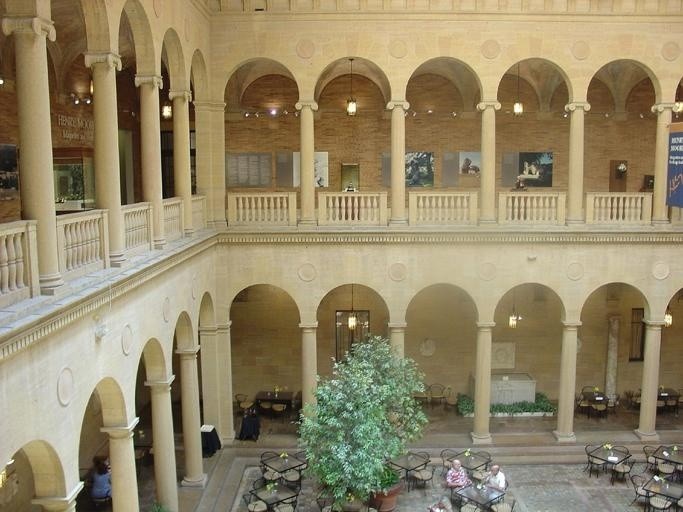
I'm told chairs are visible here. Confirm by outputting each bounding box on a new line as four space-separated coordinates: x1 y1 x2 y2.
239 448 515 512
87 441 154 512
584 442 683 511
572 384 682 423
417 380 447 410
234 392 287 420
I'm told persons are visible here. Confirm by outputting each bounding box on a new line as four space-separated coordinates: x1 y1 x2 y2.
428 495 454 512
445 459 472 493
481 464 506 492
87 455 112 499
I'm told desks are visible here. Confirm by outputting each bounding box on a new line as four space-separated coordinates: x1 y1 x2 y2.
257 390 293 411
132 426 153 451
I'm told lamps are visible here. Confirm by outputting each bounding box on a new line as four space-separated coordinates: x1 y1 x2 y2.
346 56 358 120
347 284 358 331
507 288 519 329
511 59 524 120
